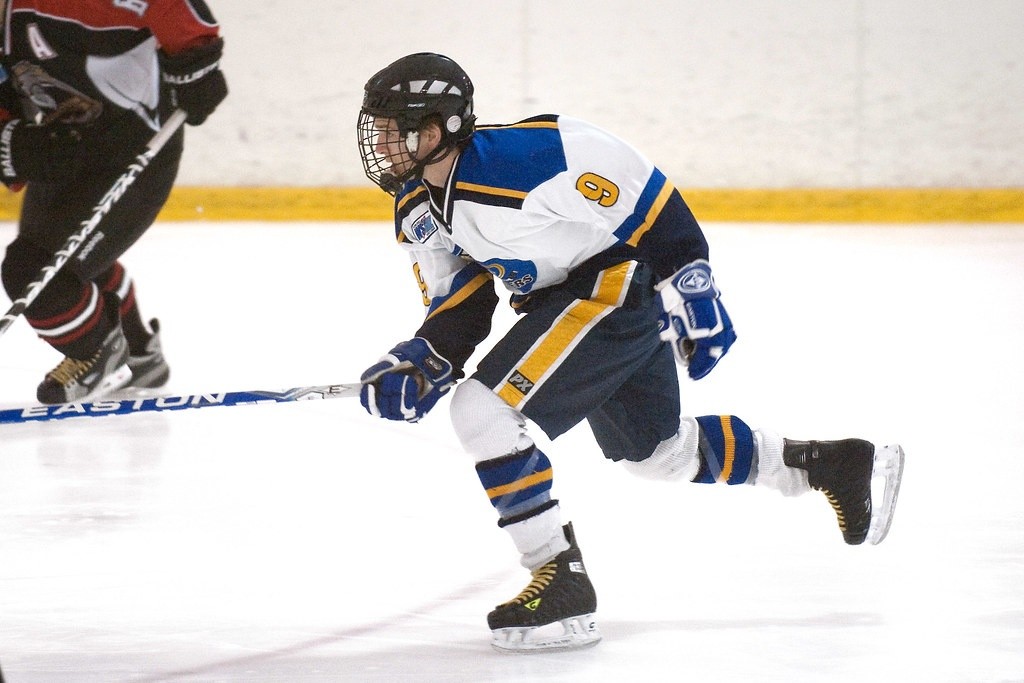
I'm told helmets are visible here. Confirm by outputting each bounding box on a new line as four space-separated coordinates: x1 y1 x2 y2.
356 52 477 194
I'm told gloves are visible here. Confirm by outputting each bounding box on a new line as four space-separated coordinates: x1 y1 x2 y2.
359 338 464 422
651 258 737 381
0 116 81 184
154 36 227 126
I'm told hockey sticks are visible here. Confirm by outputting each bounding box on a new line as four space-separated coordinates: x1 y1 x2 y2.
1 97 190 348
0 376 424 426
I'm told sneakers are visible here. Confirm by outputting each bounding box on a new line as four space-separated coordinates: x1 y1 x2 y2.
488 520 602 651
96 316 170 401
782 438 907 543
37 291 144 406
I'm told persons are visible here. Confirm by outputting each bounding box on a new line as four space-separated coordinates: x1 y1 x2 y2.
0 0 230 407
356 51 905 652
